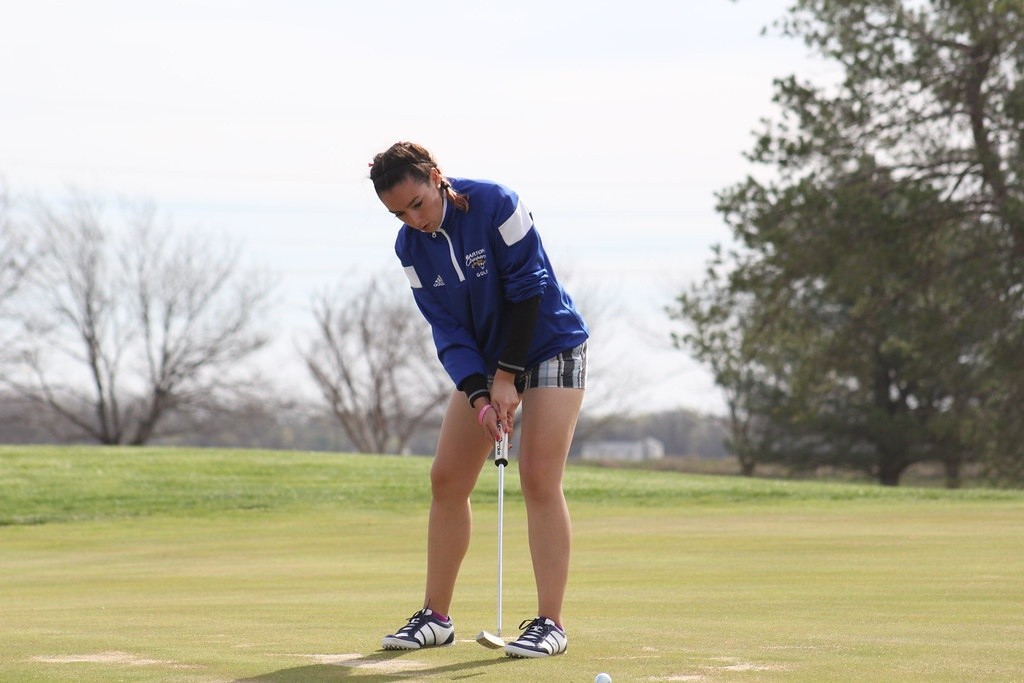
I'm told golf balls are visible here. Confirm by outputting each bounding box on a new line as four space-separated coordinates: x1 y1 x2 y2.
595 673 612 683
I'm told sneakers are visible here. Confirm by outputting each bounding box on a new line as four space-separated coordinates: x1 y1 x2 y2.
383 599 454 649
505 617 567 657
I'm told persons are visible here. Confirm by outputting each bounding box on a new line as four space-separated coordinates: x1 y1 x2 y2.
368 142 591 658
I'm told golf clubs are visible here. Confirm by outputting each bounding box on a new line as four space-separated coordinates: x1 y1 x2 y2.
475 422 509 650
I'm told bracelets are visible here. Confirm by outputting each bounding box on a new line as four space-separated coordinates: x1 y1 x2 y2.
479 405 493 426
